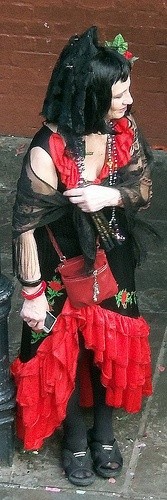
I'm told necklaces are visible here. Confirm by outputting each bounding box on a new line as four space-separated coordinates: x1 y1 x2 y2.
75 122 125 242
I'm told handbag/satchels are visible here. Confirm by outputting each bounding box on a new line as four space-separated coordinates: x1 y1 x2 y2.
57 249 119 308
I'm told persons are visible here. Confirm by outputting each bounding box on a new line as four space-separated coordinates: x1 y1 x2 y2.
11 27 154 486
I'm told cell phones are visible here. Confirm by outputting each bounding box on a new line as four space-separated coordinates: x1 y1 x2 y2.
42 310 57 333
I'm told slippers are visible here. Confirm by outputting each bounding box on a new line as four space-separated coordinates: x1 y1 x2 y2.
91 440 123 477
61 442 95 486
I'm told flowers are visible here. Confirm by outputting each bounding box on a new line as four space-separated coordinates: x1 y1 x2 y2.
104 33 139 65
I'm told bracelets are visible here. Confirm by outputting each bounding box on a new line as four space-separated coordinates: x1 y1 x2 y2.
17 275 46 300
